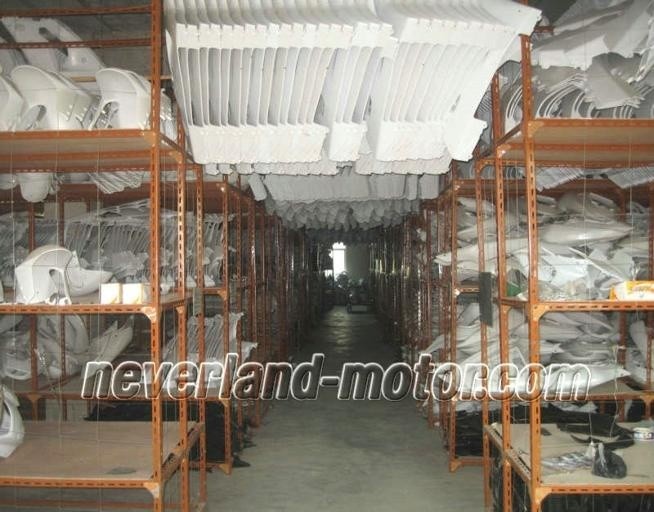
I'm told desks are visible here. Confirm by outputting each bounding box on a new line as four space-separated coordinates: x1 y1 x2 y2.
347 300 374 313
